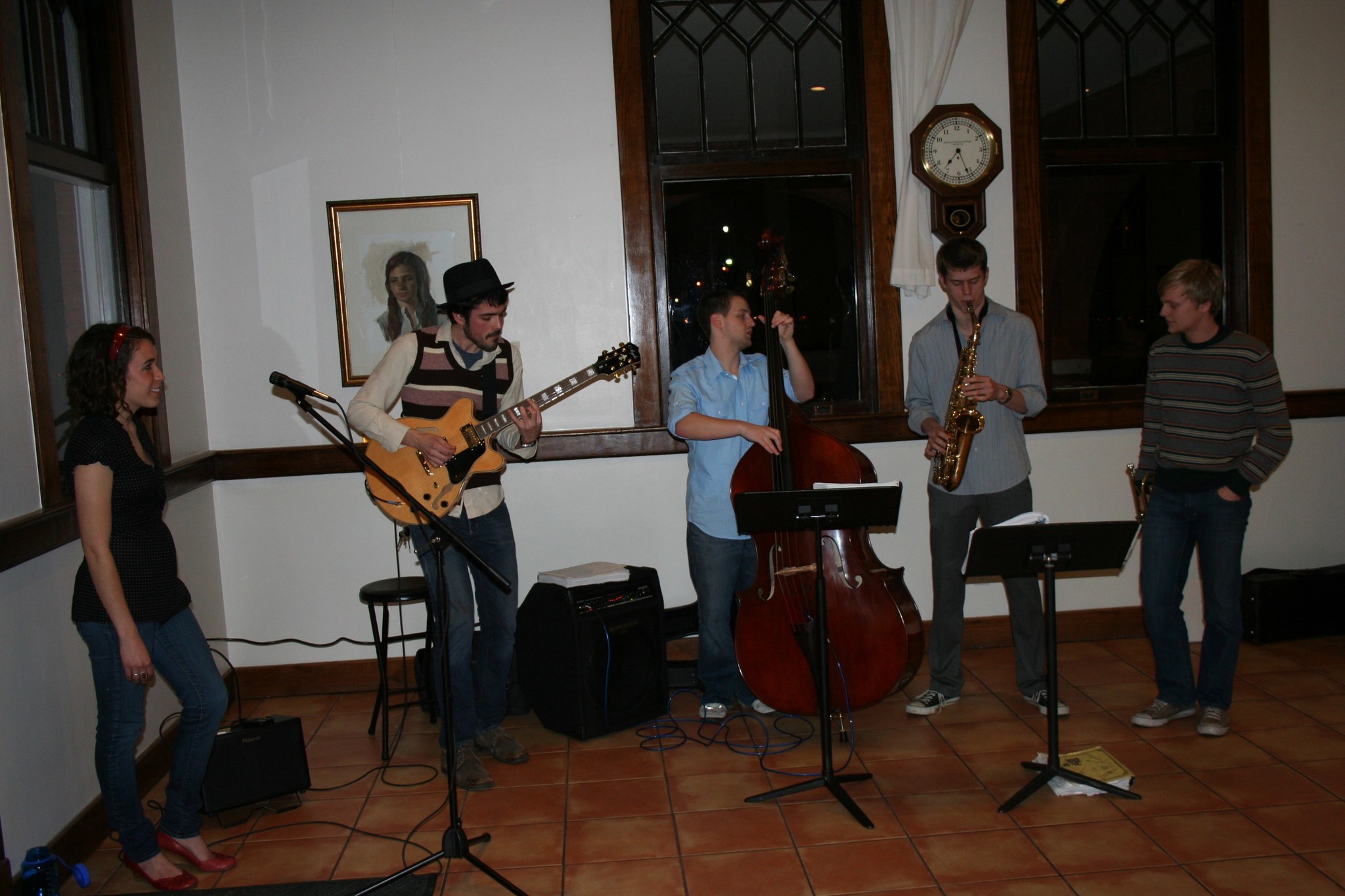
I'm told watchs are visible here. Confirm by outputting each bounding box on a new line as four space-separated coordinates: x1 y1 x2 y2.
520 435 536 448
998 386 1013 405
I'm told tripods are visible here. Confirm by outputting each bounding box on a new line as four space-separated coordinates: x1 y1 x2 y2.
963 521 1142 813
735 482 904 830
295 390 530 895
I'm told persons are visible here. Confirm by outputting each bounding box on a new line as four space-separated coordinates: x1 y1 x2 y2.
668 290 814 719
346 259 543 789
67 323 236 890
904 238 1070 715
1131 260 1294 735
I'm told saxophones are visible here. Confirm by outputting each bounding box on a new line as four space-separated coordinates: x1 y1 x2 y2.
930 301 985 492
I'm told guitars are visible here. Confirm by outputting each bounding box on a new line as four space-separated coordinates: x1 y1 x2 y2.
364 342 642 525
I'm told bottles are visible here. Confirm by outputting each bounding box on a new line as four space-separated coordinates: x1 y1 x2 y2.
21 846 91 896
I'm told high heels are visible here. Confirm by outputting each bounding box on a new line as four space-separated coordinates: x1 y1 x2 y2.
155 829 238 873
125 853 199 892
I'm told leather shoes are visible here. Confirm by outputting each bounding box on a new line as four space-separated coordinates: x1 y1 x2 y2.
473 724 530 765
439 743 495 791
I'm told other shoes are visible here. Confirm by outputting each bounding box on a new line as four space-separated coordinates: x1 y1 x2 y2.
752 699 777 715
698 703 726 723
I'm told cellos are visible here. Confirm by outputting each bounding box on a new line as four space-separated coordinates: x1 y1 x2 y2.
728 227 927 716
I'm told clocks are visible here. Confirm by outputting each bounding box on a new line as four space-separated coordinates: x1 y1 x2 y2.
910 103 1004 244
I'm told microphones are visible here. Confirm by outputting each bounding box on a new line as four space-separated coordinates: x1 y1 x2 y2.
268 371 338 405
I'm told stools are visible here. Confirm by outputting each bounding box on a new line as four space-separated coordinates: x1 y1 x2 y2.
359 577 430 762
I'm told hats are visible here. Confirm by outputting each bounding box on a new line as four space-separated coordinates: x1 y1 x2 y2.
436 258 514 308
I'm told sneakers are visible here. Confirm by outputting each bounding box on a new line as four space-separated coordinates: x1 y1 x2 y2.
1131 700 1197 728
1197 706 1235 734
905 686 962 716
1021 689 1069 717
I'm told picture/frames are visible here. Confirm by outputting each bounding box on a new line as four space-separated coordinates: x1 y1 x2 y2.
325 193 482 388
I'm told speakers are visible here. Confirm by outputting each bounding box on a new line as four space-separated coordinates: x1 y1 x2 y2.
196 714 312 815
516 565 670 740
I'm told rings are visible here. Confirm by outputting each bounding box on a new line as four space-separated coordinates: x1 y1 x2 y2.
132 673 139 676
140 672 147 679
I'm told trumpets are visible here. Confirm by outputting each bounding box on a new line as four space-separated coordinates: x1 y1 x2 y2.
1129 466 1153 531
